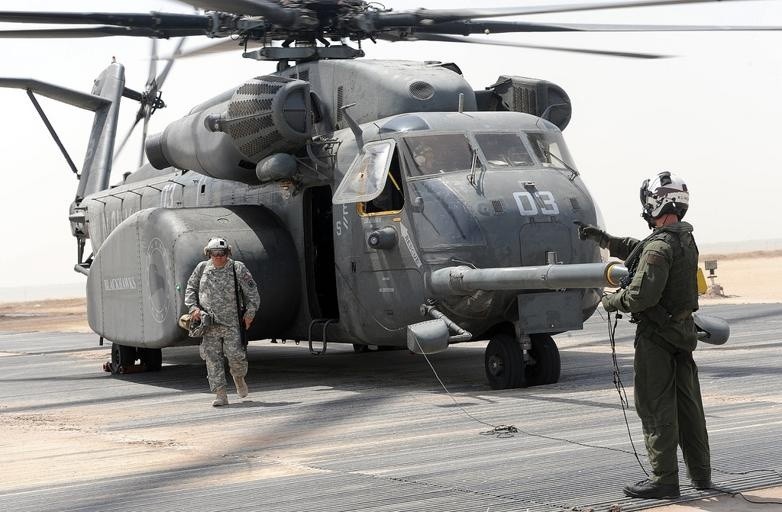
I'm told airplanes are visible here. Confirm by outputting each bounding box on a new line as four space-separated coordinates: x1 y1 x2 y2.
0 0 776 390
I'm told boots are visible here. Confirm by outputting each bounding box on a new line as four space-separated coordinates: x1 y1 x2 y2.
212 386 228 406
233 376 248 398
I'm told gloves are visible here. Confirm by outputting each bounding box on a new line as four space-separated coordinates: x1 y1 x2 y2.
601 294 615 312
573 219 600 241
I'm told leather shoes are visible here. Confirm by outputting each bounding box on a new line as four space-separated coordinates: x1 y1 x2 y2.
623 476 680 499
691 479 713 489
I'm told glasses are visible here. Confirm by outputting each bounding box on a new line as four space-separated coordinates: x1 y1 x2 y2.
211 251 226 256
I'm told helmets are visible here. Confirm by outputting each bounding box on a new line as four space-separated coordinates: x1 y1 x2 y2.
208 237 228 257
188 310 211 338
639 170 690 229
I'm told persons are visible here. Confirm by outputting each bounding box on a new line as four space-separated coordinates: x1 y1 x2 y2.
574 171 712 499
185 238 261 407
